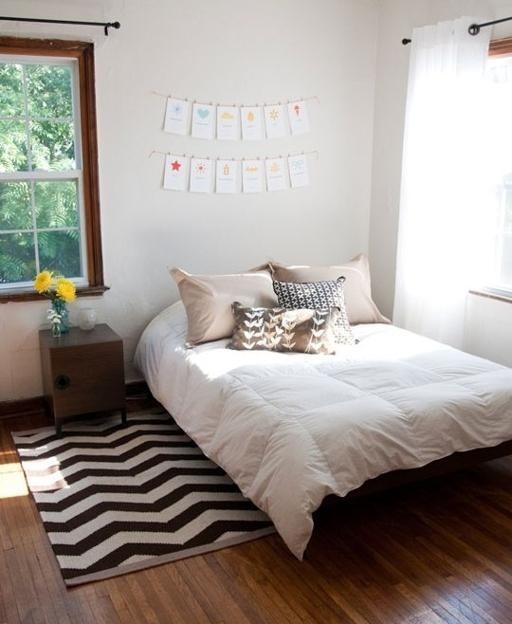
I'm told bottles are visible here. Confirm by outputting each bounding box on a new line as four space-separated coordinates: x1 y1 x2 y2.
79 307 99 329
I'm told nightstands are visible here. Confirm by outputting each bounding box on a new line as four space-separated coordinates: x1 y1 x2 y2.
48 338 127 436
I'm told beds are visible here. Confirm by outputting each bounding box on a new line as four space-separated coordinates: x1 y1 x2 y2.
131 264 512 538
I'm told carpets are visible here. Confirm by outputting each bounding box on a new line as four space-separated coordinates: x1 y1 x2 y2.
6 402 283 591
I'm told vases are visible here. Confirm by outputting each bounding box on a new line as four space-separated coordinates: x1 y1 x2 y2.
51 298 74 338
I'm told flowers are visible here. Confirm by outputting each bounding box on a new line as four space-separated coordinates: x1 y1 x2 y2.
32 270 78 325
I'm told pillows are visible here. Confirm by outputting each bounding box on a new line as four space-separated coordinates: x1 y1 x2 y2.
164 253 397 360
